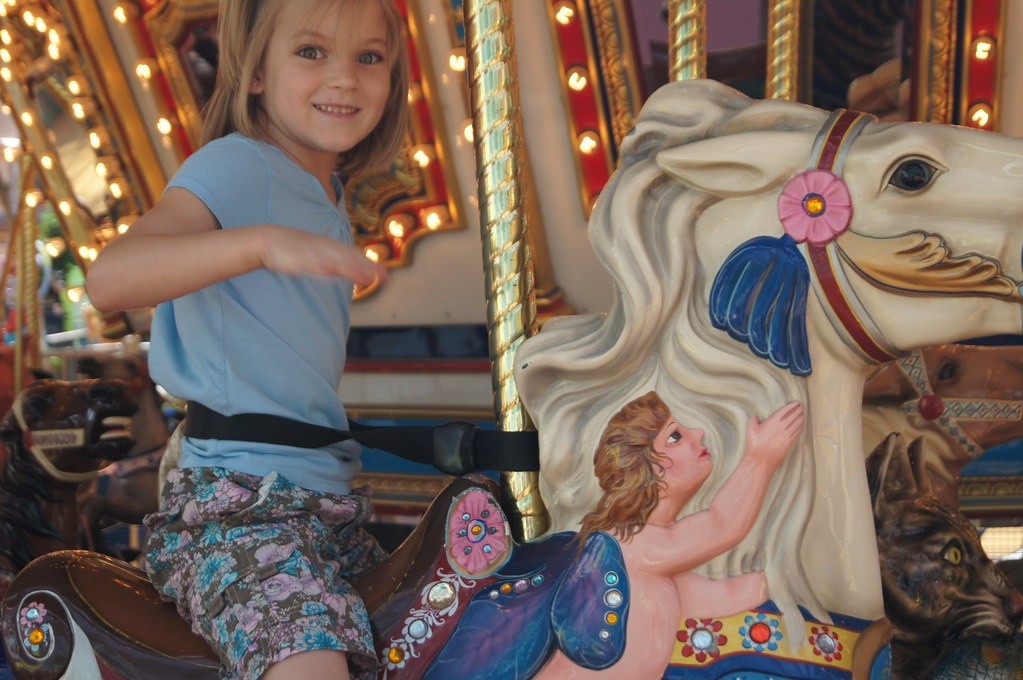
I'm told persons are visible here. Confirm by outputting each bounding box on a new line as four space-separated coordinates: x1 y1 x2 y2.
39 225 73 303
87 0 408 679
530 391 804 680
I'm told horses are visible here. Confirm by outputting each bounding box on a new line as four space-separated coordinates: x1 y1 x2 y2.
1 80 1023 680
